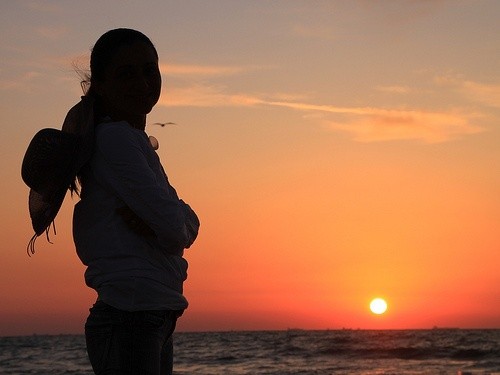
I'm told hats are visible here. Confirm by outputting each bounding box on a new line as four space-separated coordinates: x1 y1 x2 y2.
20 102 89 236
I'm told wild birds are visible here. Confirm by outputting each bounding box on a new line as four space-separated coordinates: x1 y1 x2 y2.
154 122 177 127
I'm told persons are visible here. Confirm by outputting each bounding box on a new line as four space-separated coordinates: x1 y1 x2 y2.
19 26 200 374
